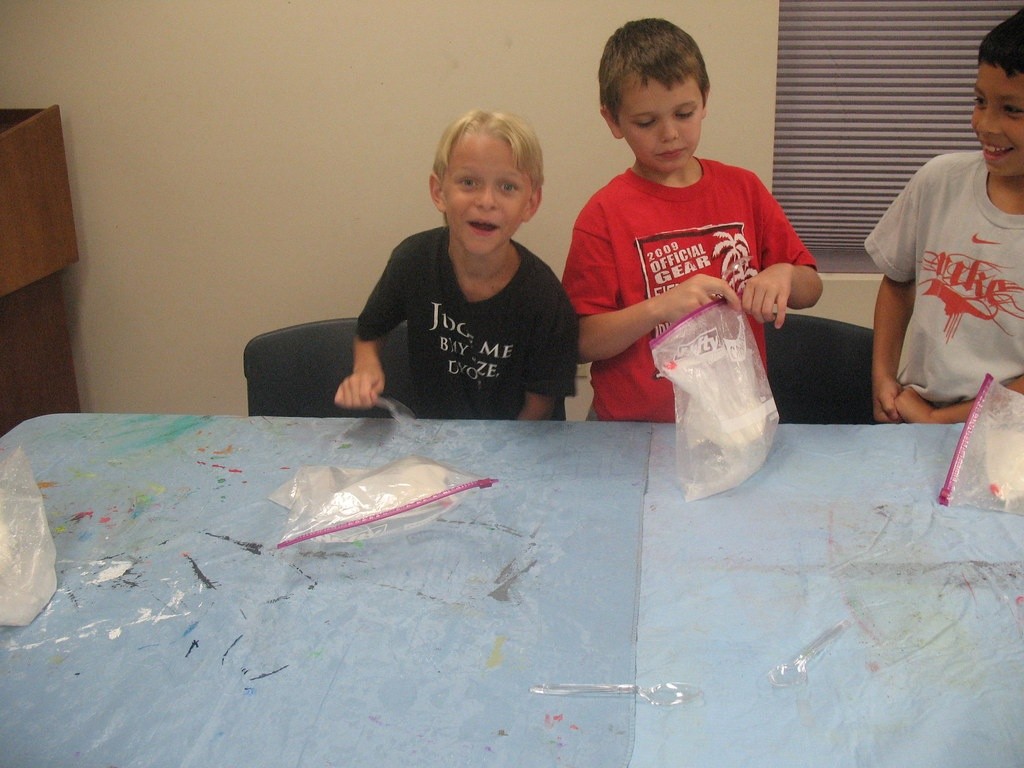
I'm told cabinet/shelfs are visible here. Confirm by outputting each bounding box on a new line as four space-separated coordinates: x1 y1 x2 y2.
1 103 80 435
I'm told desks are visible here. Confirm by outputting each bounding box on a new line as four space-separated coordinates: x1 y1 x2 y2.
0 411 1024 768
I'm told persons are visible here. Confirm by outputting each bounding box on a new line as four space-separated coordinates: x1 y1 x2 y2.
856 6 1023 424
335 107 580 421
561 17 823 421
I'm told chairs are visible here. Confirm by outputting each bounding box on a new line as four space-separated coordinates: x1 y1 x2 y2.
764 310 876 425
242 315 421 422
